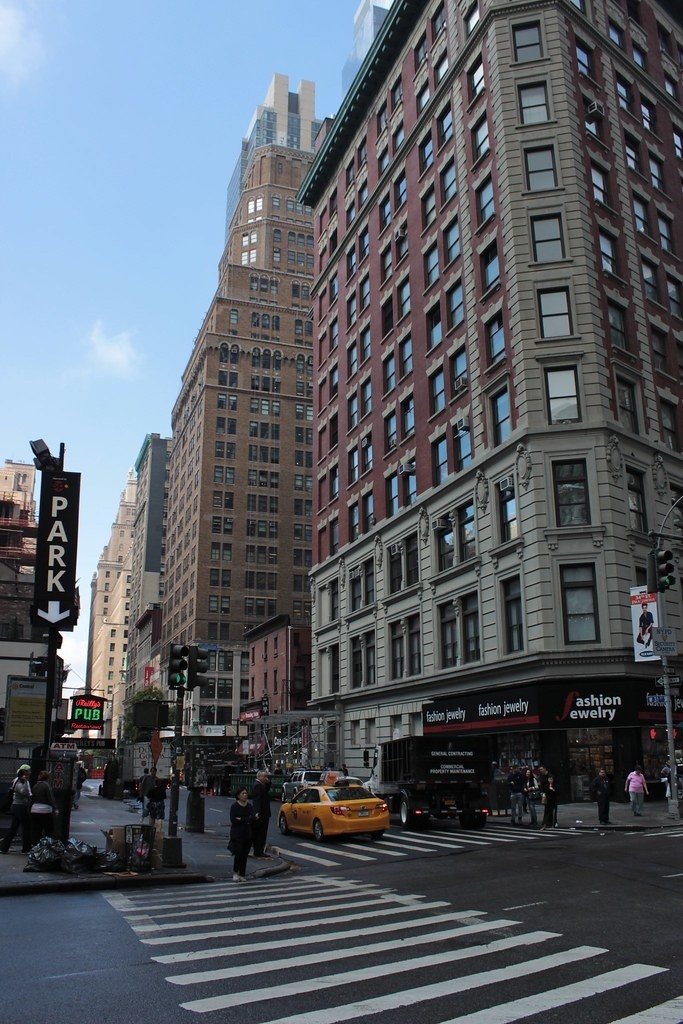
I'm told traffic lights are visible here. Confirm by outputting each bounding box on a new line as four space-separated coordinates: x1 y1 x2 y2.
189 646 209 686
648 726 658 741
168 644 188 686
657 549 675 589
672 727 678 739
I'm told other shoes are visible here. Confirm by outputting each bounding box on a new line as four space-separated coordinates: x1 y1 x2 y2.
74 803 80 809
510 820 515 826
600 820 612 825
21 849 31 854
518 816 523 824
633 811 641 816
254 852 271 857
528 821 538 826
232 874 246 882
137 816 144 824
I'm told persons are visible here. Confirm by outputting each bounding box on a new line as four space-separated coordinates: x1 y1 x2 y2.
660 760 670 787
338 763 348 776
146 778 167 832
74 763 87 809
590 768 611 825
273 764 283 774
230 786 255 882
506 764 558 830
625 765 649 816
492 761 504 774
252 771 272 857
138 766 158 822
637 603 654 648
0 764 59 854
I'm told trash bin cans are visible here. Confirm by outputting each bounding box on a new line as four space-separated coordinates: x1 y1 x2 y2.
123 823 157 873
186 797 206 833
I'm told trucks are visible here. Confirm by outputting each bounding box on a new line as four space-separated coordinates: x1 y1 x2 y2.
362 735 490 828
113 742 172 797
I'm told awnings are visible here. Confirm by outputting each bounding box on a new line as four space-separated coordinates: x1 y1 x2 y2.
235 739 271 755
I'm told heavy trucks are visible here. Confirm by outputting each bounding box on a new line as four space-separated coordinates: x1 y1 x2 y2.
183 744 243 796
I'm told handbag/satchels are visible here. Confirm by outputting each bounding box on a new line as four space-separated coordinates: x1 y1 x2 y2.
138 783 144 797
528 790 542 802
3 789 13 811
540 793 547 804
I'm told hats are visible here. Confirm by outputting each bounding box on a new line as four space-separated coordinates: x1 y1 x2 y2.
666 760 671 765
144 768 149 773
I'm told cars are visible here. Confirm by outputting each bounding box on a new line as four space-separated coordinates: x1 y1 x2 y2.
277 783 390 842
334 776 364 787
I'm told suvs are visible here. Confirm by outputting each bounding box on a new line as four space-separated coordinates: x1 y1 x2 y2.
280 771 345 804
